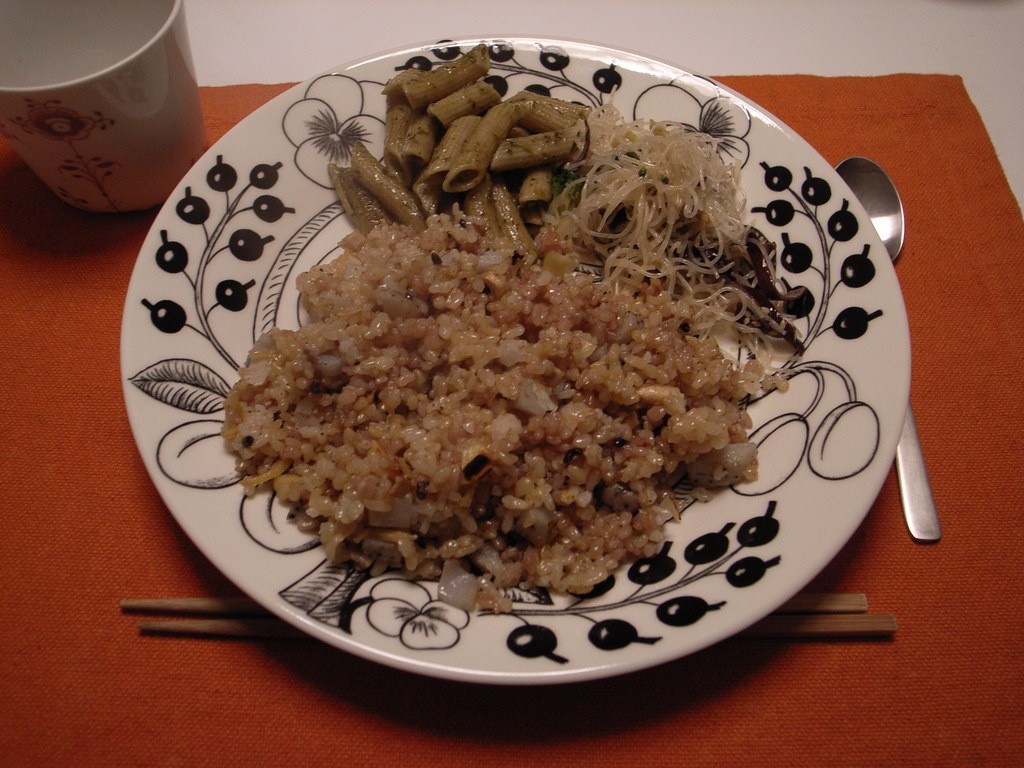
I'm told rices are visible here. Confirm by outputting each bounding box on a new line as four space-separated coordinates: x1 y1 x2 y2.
218 206 789 612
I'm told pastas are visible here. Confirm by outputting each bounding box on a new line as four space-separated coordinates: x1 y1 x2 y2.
327 39 586 257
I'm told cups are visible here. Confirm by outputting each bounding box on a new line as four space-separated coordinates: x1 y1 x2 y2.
0 0 208 214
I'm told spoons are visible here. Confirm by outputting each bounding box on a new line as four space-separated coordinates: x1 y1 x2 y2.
834 156 940 543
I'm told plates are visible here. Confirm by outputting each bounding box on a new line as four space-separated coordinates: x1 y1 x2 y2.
117 35 913 688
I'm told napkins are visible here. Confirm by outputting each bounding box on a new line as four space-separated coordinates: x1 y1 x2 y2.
0 78 1024 768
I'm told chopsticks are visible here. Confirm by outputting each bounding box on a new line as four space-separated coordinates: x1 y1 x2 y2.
120 587 896 658
